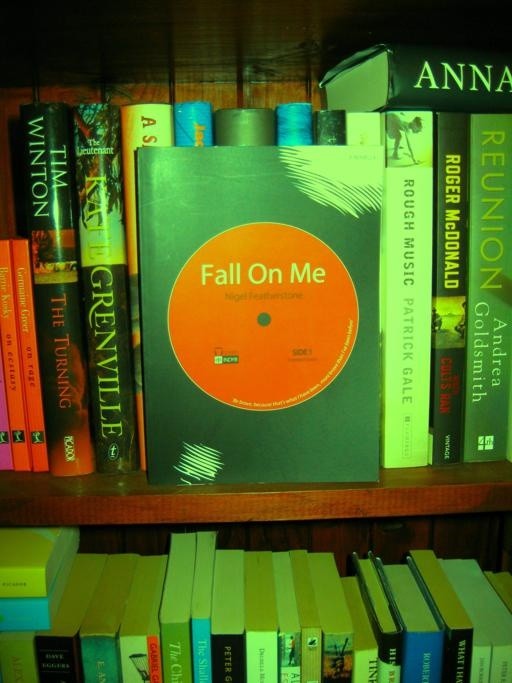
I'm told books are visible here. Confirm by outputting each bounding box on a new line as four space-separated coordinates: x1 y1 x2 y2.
1 526 512 681
0 36 512 486
